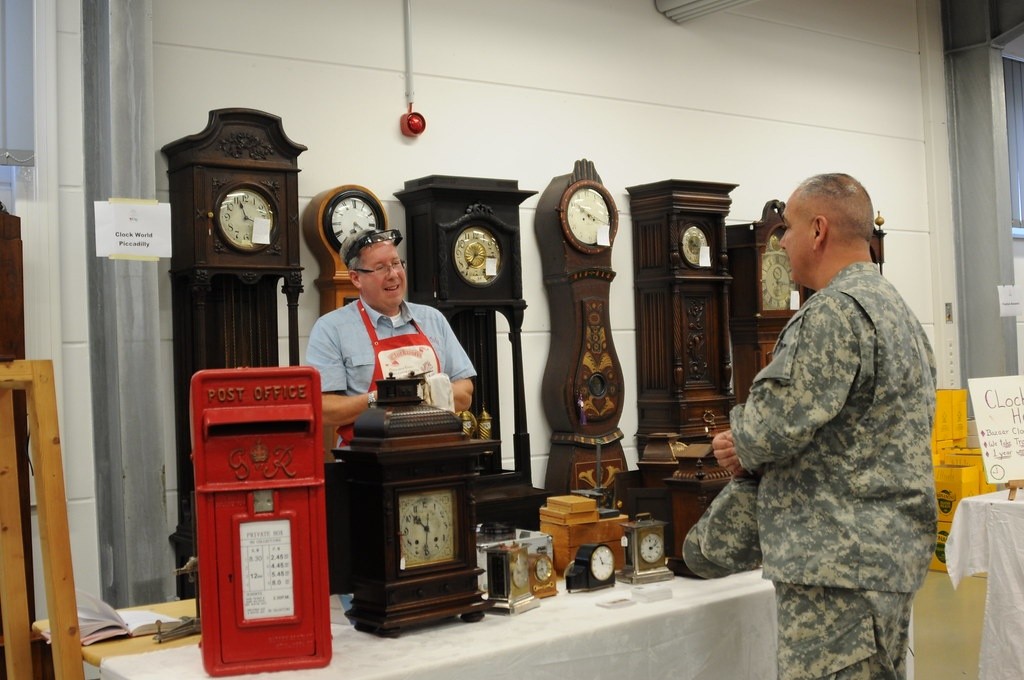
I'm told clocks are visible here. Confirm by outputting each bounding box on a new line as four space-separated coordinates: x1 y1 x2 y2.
334 373 509 638
735 196 808 403
564 544 618 591
391 175 540 515
621 519 672 583
486 548 545 612
540 159 629 499
624 173 740 455
161 108 305 597
529 553 558 597
302 185 388 320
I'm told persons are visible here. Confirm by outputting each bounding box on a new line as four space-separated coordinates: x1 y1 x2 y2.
711 172 937 680
304 229 478 464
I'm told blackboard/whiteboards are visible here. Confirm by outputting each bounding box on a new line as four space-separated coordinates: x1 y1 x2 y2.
966 375 1024 485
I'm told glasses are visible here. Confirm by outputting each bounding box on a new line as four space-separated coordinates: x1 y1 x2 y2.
356 259 406 276
344 228 403 268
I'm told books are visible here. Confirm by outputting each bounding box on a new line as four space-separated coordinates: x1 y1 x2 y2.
40 589 183 646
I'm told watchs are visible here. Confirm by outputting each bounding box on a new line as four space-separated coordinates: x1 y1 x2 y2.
367 390 377 408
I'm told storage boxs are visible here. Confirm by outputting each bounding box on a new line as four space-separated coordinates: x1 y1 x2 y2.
540 495 627 577
921 390 995 577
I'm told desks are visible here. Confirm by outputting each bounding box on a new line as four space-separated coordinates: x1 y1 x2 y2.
31 570 914 680
945 488 1023 680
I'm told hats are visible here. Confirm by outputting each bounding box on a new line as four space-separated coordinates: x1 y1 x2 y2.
683 475 763 578
